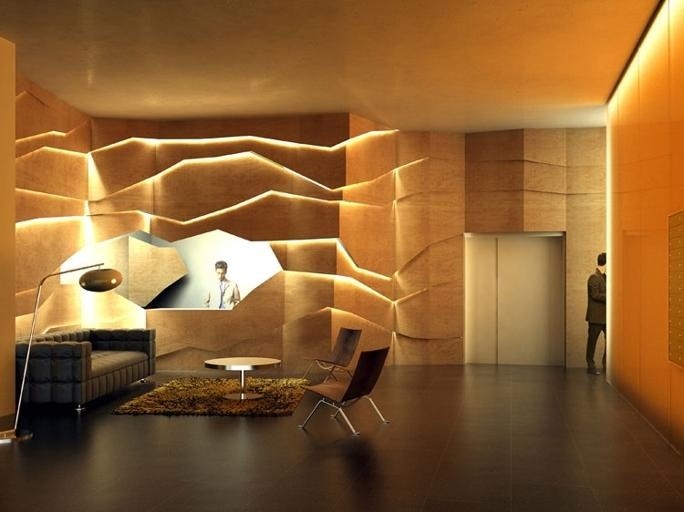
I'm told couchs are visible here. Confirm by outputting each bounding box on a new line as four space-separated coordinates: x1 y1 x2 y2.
13 326 158 411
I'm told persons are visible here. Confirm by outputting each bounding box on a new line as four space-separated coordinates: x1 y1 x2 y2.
199 258 240 310
584 252 607 375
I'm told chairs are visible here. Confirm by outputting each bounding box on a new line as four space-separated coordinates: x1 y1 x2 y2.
297 345 390 436
300 328 362 385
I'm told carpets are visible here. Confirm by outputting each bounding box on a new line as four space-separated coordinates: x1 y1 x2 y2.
107 374 310 419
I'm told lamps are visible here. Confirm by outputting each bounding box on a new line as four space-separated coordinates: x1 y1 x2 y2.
1 263 123 441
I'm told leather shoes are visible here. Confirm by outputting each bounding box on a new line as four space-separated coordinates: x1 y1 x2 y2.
587 370 600 375
